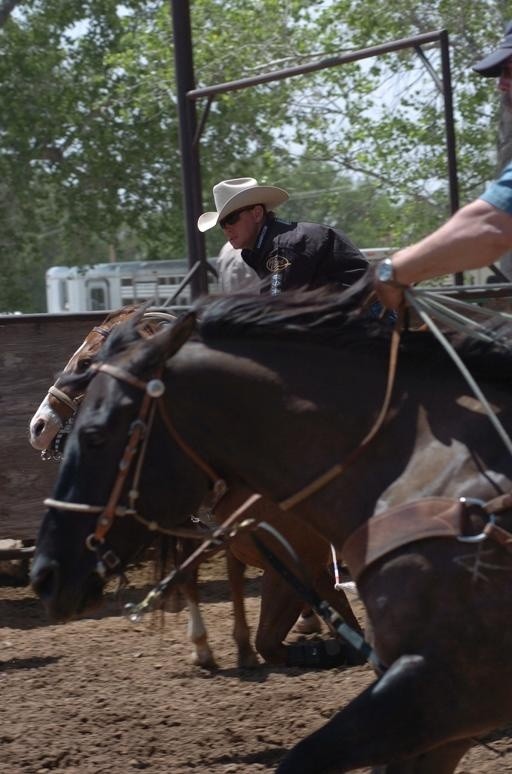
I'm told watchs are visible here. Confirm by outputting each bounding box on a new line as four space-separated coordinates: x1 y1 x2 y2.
376 257 411 290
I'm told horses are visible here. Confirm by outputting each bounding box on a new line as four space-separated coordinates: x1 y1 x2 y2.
25 280 510 772
27 299 342 671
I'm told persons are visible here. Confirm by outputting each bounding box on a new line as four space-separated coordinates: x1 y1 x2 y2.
373 23 512 310
216 241 262 297
197 177 369 290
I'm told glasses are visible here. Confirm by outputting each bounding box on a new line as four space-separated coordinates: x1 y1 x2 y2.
219 210 242 228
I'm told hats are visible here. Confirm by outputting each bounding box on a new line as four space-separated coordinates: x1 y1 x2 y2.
473 21 511 77
197 178 289 233
